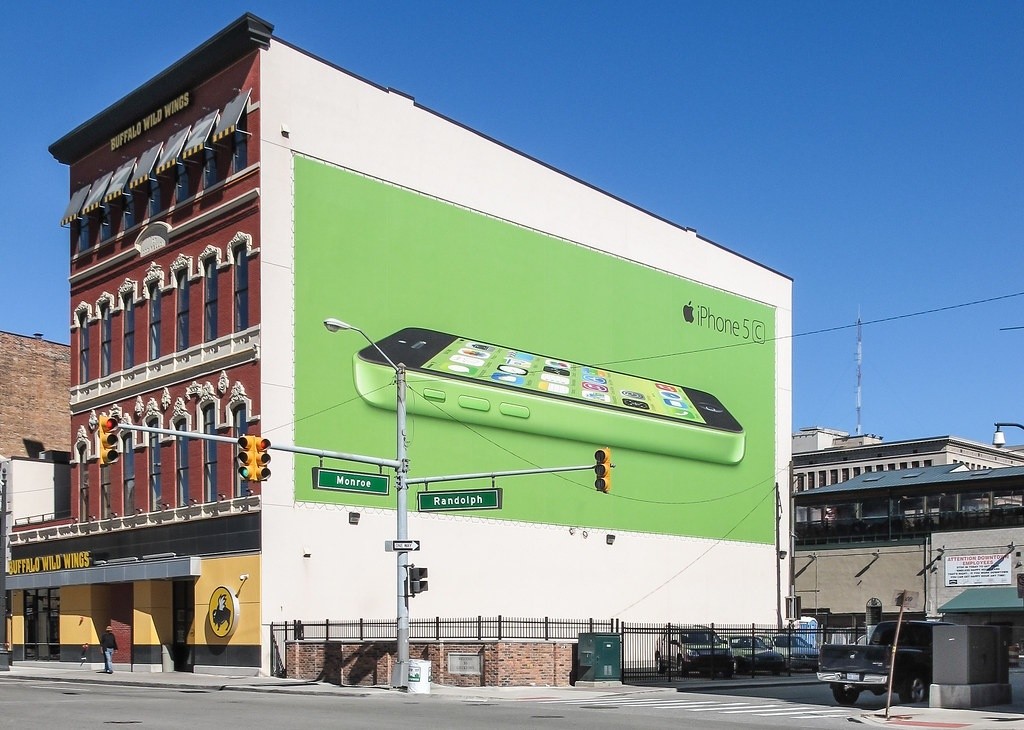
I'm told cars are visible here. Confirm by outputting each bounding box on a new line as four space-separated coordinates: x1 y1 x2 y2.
755 633 819 674
718 634 786 678
850 634 868 645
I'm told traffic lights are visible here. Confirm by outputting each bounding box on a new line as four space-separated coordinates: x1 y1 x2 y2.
594 449 610 494
236 435 272 482
98 415 119 468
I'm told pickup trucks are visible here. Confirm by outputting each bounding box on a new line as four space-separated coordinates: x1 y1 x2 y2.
816 621 960 704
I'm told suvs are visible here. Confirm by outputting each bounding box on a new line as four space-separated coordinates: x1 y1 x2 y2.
654 624 735 680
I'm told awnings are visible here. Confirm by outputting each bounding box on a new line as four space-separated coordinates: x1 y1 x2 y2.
182 108 219 160
212 87 253 142
130 141 164 189
61 183 92 226
82 170 114 215
155 124 191 174
104 156 138 202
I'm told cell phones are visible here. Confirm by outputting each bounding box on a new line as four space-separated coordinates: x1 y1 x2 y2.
352 328 744 466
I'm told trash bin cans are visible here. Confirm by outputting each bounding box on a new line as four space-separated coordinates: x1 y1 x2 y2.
407 659 432 694
162 644 175 672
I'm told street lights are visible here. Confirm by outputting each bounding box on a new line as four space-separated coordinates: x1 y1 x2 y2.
809 553 819 618
990 417 1024 451
324 318 412 691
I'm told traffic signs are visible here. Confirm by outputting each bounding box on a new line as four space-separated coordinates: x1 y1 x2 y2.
317 469 389 496
421 492 497 510
393 539 420 551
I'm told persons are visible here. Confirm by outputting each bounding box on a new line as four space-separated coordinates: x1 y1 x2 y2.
100 626 118 674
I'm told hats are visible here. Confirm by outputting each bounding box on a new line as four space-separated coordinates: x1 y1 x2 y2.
106 626 112 630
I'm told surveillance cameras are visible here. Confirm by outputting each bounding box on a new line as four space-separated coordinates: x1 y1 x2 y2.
993 431 1005 448
240 576 244 581
